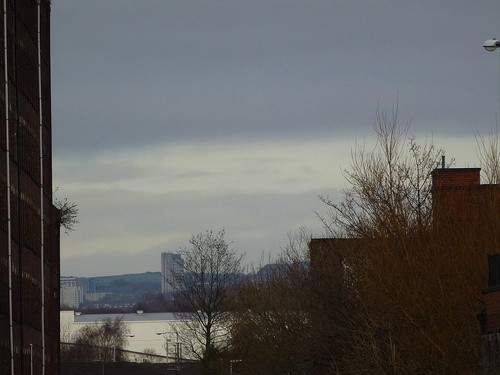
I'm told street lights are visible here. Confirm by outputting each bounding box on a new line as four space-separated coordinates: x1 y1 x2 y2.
114 334 135 363
156 332 182 364
